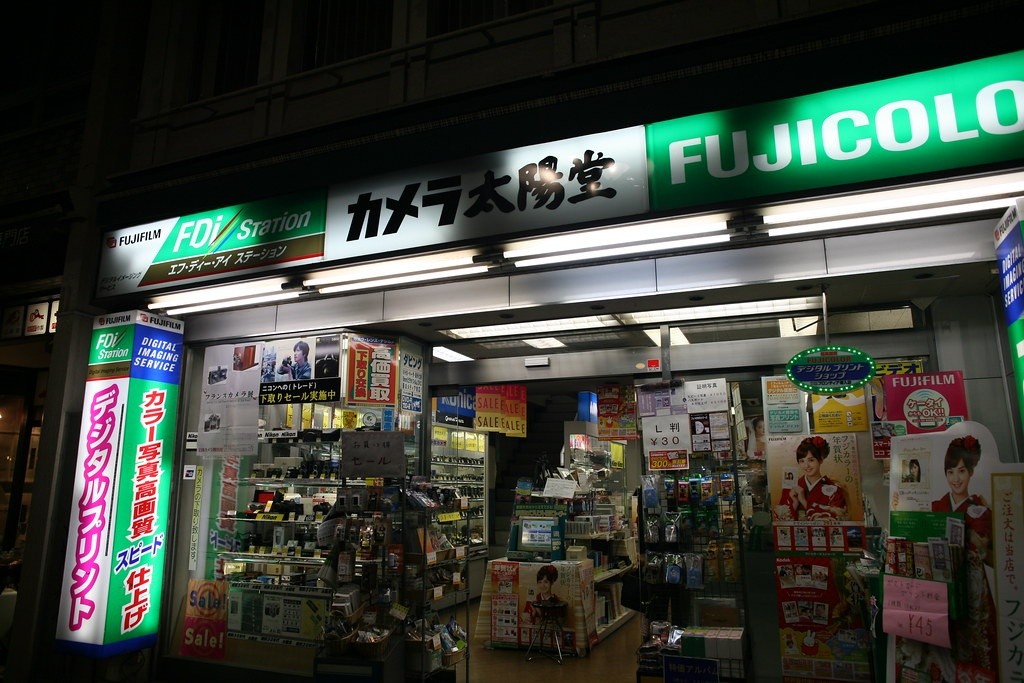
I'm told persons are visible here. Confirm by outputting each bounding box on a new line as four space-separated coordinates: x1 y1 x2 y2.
524 566 564 625
753 495 770 519
779 528 841 653
745 416 766 458
779 436 849 520
932 434 996 570
904 459 921 482
528 590 534 596
786 472 794 481
262 341 311 382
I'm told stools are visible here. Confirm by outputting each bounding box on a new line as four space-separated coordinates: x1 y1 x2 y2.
524 600 576 665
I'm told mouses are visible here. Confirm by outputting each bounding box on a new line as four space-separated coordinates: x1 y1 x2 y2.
534 556 544 561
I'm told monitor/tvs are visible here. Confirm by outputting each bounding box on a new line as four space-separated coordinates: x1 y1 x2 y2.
517 516 559 558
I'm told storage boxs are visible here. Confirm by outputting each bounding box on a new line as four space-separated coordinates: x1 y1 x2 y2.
704 626 720 658
728 627 748 673
405 649 442 674
312 634 405 683
716 627 730 673
565 546 588 561
692 626 709 658
681 625 697 657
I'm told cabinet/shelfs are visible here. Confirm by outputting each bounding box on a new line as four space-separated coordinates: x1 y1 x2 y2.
401 477 471 683
564 421 637 642
204 437 418 567
262 397 490 611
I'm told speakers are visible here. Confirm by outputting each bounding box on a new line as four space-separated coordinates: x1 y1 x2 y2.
506 523 532 561
